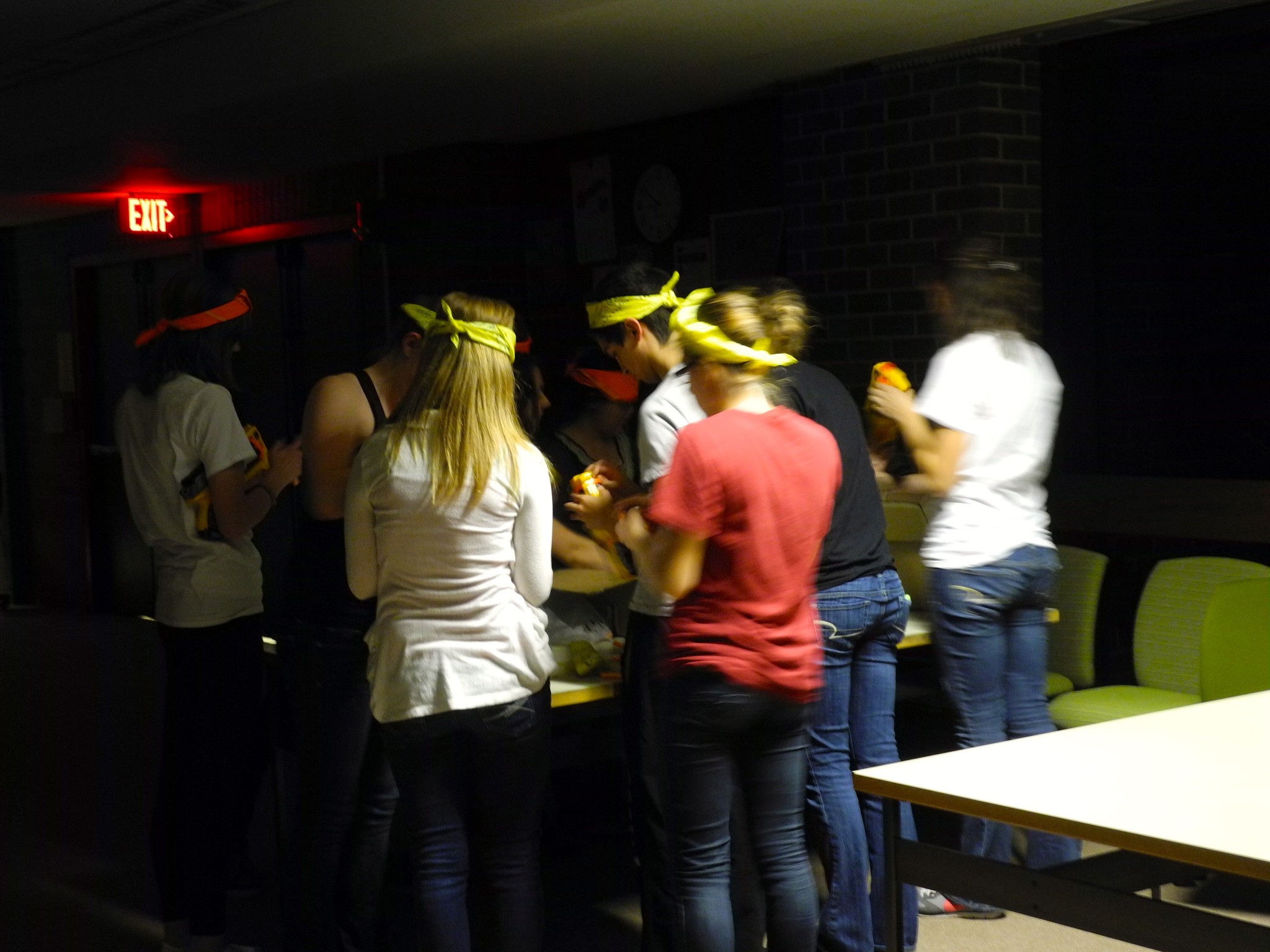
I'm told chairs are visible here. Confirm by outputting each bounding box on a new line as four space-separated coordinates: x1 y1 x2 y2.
1036 543 1270 735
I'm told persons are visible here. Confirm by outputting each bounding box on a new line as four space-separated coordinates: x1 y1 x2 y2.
865 247 1082 870
114 270 302 952
616 289 842 952
344 295 555 952
300 293 443 951
511 262 708 952
768 361 917 951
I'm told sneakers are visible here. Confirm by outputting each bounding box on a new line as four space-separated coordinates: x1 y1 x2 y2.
918 889 1006 920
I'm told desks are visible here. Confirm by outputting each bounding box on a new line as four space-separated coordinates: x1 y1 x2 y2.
547 605 1059 952
855 689 1270 952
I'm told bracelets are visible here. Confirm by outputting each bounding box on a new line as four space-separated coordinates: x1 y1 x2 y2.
245 483 274 504
